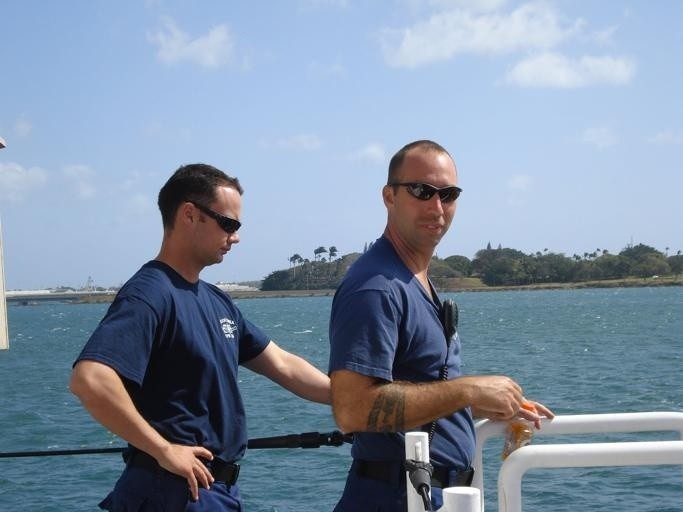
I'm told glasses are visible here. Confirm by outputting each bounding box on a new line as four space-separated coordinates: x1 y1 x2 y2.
387 180 463 204
184 198 242 235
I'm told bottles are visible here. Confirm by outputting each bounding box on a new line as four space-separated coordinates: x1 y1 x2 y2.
500 402 539 462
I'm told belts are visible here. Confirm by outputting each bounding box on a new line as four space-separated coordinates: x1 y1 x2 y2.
206 460 240 486
429 467 475 488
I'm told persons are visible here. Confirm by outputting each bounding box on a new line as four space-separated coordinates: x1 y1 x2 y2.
67 163 333 511
328 138 556 512
